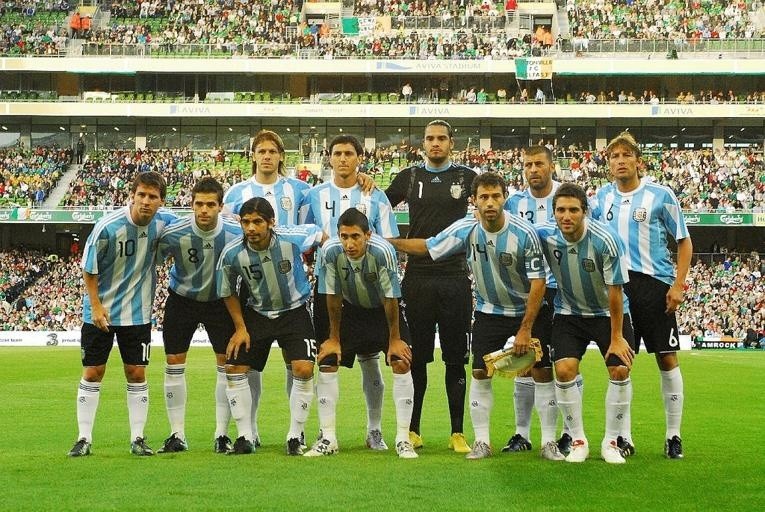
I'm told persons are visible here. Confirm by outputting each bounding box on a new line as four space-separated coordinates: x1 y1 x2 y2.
1 120 763 348
289 133 406 455
219 124 324 456
496 140 584 461
0 0 764 60
382 172 568 463
519 179 630 463
62 171 243 460
400 77 762 103
155 175 239 457
379 121 493 461
580 127 697 464
210 195 321 459
313 205 423 462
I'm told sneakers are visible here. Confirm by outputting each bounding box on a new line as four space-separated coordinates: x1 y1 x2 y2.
664 436 683 459
566 439 590 462
286 434 303 455
303 438 339 456
395 440 418 458
409 431 423 448
618 436 635 457
601 438 626 464
557 433 572 454
300 433 307 450
540 441 566 460
366 430 388 450
215 434 260 455
448 433 471 453
129 437 154 456
68 437 92 456
466 441 492 459
156 434 188 453
501 434 532 452
